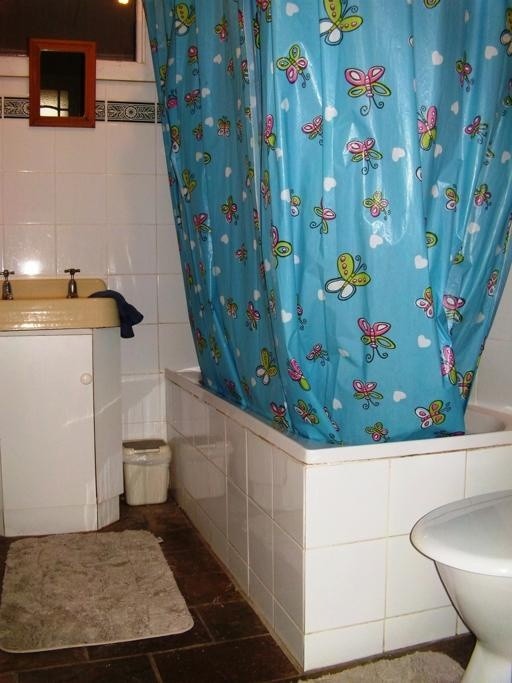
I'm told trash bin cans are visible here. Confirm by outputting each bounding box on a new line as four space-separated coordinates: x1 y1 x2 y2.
122 438 171 506
172 435 233 501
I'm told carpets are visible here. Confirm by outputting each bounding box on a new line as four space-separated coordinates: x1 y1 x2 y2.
1 530 193 655
287 650 466 682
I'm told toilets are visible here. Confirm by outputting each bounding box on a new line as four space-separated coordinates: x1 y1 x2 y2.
408 487 511 682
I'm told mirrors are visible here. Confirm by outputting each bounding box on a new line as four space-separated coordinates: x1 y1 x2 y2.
29 38 96 128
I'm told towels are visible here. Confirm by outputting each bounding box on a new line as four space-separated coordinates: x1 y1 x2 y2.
88 289 142 339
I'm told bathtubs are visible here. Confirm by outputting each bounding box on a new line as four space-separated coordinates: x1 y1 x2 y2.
165 359 512 675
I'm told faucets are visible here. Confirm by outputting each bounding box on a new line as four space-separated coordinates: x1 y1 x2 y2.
0 270 16 300
63 268 82 299
